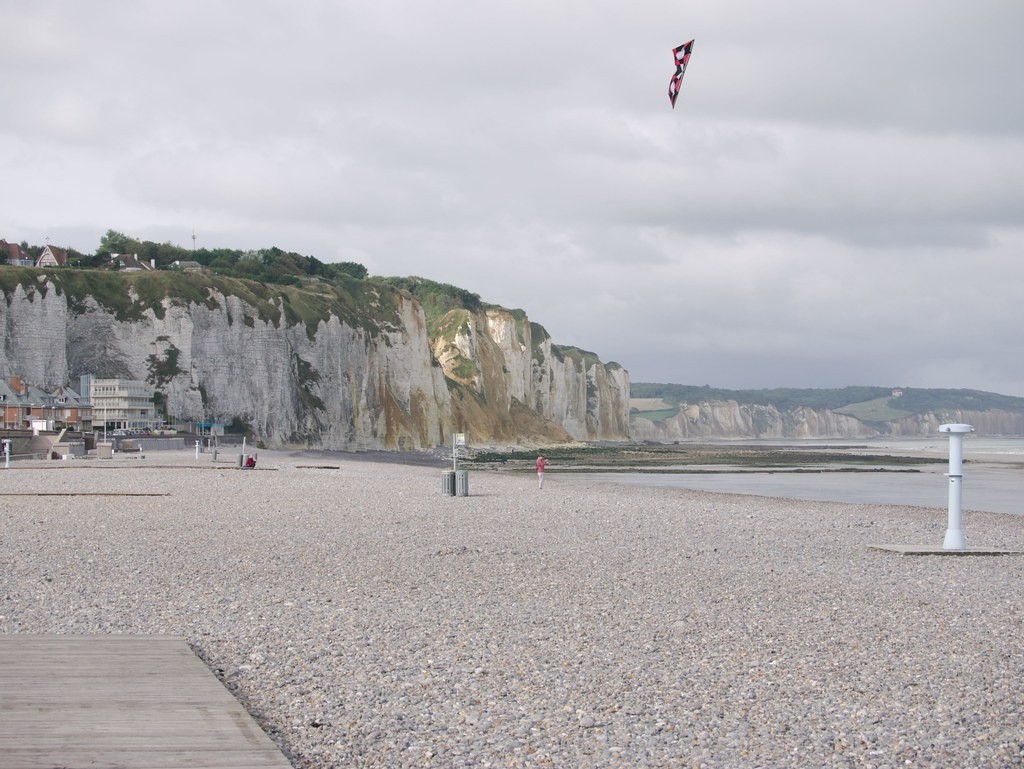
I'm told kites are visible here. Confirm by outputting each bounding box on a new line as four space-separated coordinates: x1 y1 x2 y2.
668 36 697 110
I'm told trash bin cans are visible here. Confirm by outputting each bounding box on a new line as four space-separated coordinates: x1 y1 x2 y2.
455 470 469 497
440 470 456 497
212 449 217 460
236 454 243 467
242 454 249 465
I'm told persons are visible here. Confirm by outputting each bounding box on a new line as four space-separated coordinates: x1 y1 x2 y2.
536 454 550 490
246 454 257 469
